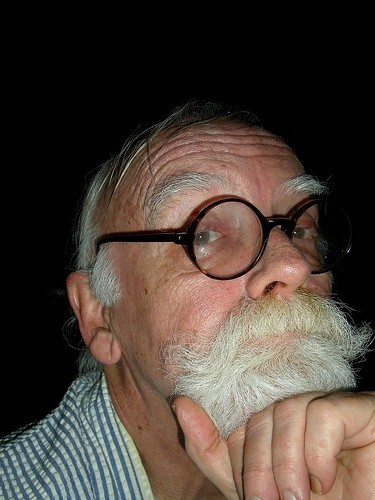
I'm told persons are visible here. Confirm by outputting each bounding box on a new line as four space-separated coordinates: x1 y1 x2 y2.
1 99 375 500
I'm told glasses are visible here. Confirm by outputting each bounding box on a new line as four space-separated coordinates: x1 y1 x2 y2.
94 191 358 280
